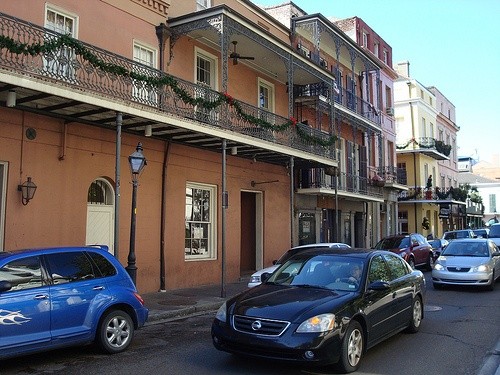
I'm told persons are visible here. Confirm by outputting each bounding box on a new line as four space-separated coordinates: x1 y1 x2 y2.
335 262 362 284
475 245 487 255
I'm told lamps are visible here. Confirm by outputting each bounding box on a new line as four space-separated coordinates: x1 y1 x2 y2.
18 176 38 206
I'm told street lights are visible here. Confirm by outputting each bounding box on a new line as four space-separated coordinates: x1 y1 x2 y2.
124 139 147 287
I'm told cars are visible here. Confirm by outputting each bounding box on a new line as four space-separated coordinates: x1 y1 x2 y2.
210 246 427 374
370 231 437 272
247 242 352 290
0 244 149 362
427 238 450 258
442 221 500 248
430 237 500 292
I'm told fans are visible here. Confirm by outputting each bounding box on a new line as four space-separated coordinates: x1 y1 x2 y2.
226 40 256 66
